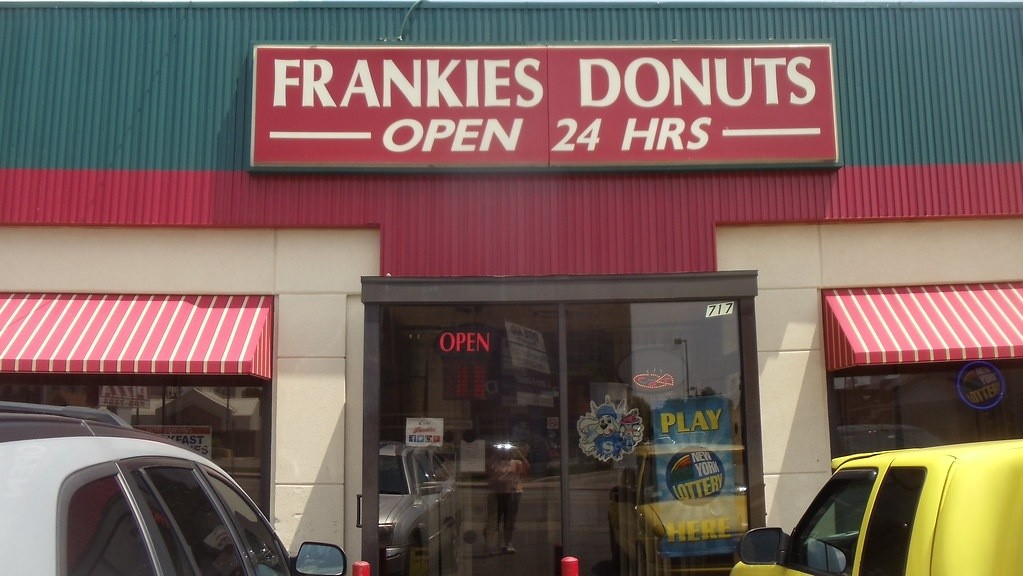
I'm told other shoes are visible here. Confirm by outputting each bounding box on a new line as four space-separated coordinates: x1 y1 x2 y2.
504 544 516 553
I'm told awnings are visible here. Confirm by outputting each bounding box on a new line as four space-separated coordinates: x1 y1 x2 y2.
0 292 271 379
820 281 1023 371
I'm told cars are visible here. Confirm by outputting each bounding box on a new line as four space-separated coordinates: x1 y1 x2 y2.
377 441 460 576
1 403 350 576
730 438 1022 576
607 442 748 575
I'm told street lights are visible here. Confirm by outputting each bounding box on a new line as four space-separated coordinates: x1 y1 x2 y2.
673 338 690 399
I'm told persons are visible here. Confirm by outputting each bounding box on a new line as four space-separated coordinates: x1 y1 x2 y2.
491 446 529 554
